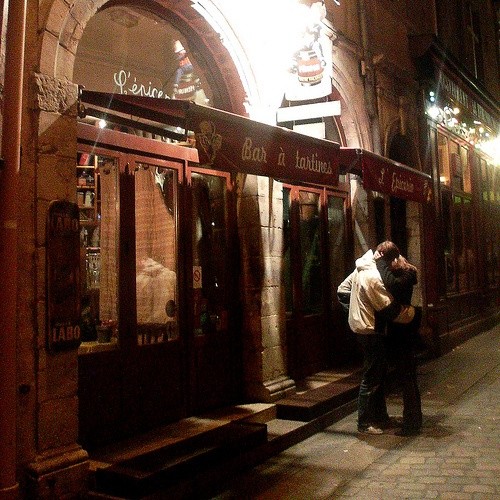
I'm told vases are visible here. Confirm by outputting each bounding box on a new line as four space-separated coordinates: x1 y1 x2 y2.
96 326 112 343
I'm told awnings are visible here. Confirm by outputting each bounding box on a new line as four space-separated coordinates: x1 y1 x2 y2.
84 91 433 212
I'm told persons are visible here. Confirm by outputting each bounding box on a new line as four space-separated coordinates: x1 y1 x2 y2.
337 241 424 437
136 258 176 324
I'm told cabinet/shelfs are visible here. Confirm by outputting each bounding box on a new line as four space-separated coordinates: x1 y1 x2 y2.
76 151 101 251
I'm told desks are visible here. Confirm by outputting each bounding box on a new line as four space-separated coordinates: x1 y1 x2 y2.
77 336 118 355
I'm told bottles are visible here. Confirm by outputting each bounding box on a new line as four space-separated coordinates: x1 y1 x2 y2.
78 153 100 289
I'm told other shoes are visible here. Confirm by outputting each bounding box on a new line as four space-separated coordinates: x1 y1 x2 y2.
380 419 400 428
362 426 385 435
395 429 421 437
